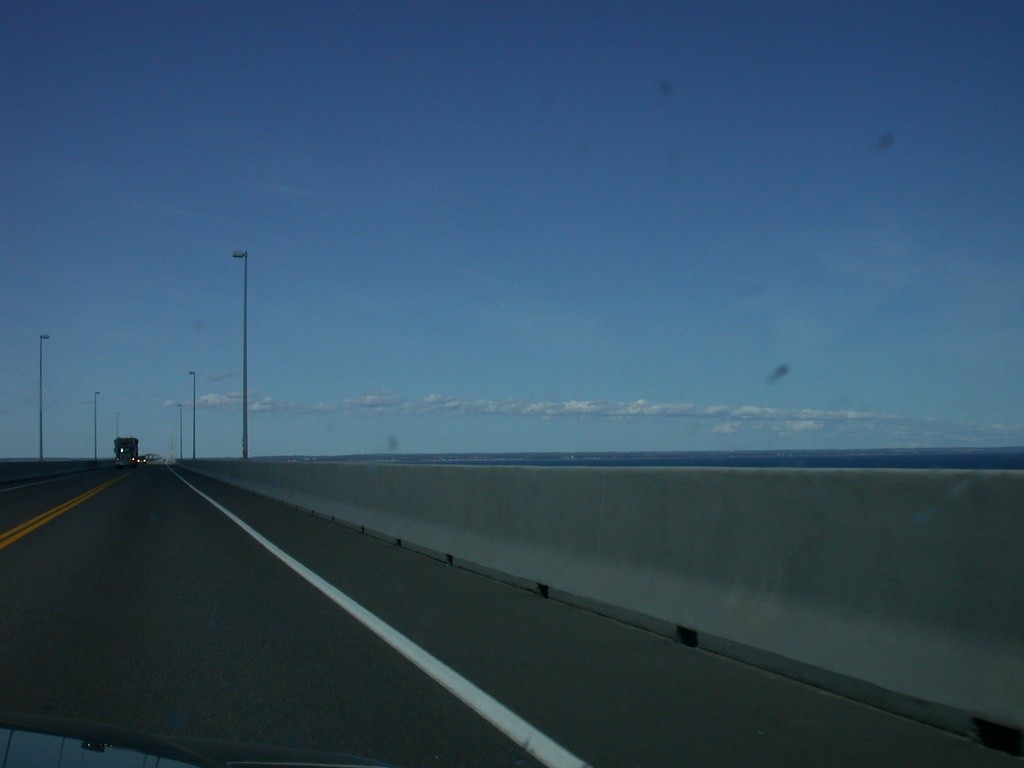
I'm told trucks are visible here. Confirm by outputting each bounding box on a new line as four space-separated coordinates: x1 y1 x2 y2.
113 437 139 468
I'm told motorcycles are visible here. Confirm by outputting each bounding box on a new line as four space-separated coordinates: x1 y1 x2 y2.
163 456 166 463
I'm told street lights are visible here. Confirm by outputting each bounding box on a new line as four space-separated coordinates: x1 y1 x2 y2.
177 403 183 460
39 334 50 459
188 370 196 460
116 412 121 439
94 391 100 460
231 248 249 459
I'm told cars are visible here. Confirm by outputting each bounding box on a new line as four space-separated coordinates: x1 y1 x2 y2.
137 456 147 464
